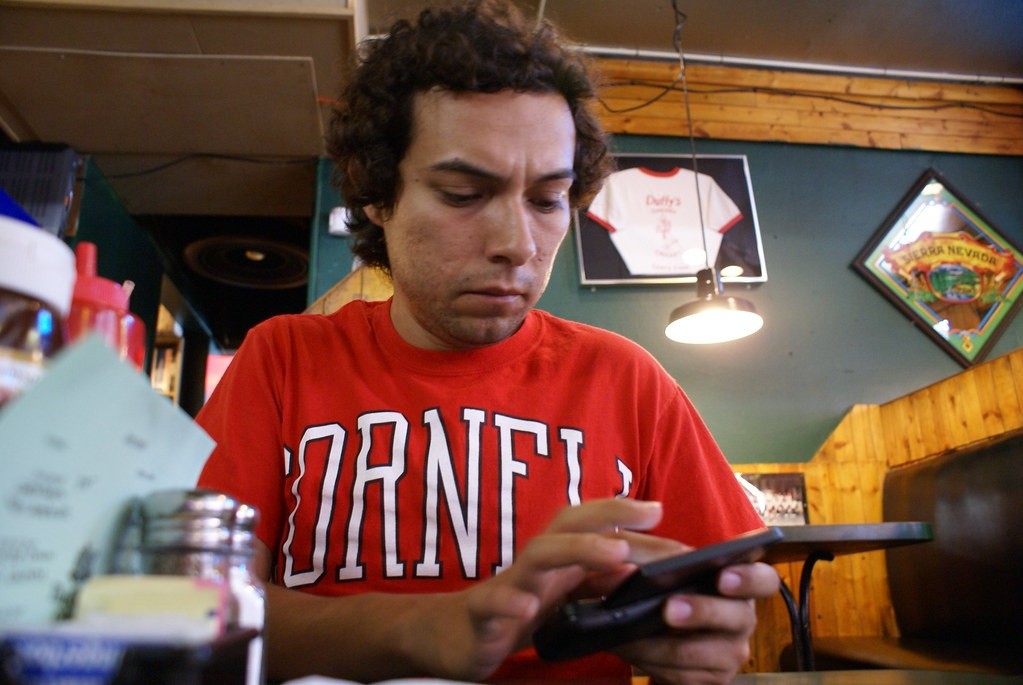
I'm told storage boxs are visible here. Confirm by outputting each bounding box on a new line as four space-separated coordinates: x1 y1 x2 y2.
74 572 224 622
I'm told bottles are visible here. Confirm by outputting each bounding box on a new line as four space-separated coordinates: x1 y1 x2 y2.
100 491 263 684
1 216 78 416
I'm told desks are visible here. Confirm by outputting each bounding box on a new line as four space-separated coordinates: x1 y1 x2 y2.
760 521 933 671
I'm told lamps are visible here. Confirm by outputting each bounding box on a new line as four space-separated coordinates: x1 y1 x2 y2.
664 0 764 344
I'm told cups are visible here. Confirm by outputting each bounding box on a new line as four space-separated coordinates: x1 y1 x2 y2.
63 243 128 357
126 311 146 365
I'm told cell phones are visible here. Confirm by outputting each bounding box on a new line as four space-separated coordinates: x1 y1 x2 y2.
526 530 783 664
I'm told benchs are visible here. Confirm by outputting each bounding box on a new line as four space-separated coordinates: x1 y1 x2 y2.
779 430 1023 671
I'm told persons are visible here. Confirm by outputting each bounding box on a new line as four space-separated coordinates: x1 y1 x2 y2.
194 0 780 685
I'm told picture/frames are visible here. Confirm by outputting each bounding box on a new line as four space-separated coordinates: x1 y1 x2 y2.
573 152 768 284
846 165 1023 370
741 471 810 525
